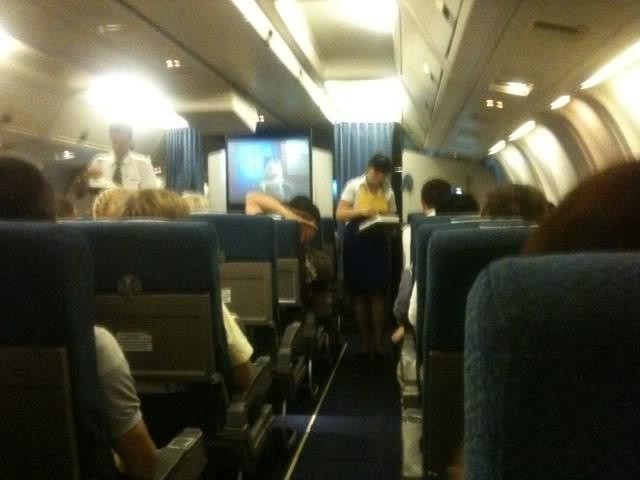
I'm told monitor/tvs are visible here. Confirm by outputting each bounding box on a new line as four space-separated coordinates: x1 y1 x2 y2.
226 128 312 214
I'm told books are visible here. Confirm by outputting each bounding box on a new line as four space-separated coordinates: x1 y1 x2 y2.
358 213 400 232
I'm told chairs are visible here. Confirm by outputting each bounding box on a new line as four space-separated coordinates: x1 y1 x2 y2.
420 225 539 478
58 214 348 469
397 215 540 404
461 250 640 479
0 220 205 479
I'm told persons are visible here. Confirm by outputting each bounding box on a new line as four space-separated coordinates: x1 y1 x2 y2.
54 187 255 389
246 191 341 357
335 153 398 357
392 177 551 343
446 158 639 479
2 150 159 479
84 123 158 189
256 157 294 205
